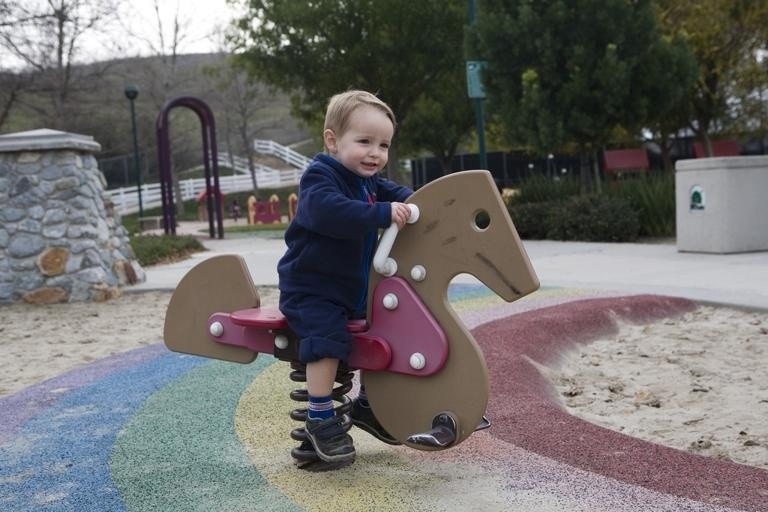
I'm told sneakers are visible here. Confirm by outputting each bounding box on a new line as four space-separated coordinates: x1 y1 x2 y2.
303 412 356 463
345 396 402 446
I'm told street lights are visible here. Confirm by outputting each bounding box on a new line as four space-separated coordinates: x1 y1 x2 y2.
123 81 144 233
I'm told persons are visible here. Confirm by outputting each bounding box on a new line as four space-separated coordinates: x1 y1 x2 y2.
274 89 418 465
